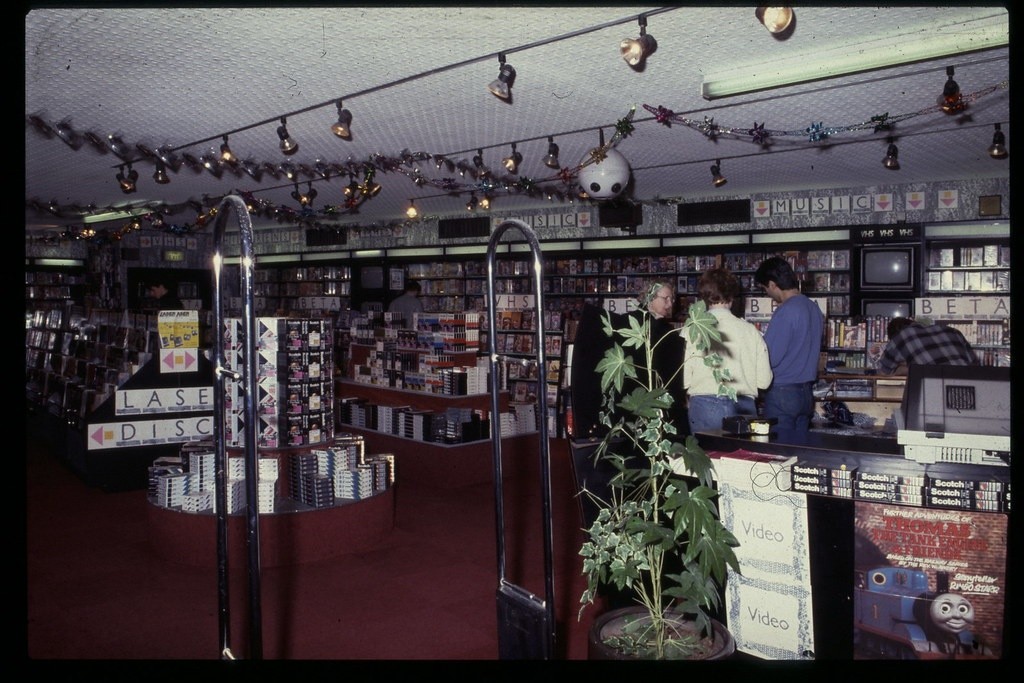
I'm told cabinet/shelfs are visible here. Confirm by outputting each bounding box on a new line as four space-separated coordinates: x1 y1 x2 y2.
28 253 1011 591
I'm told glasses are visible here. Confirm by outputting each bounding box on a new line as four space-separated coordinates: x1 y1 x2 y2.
656 294 675 303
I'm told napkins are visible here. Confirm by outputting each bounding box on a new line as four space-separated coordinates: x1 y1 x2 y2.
290 182 318 207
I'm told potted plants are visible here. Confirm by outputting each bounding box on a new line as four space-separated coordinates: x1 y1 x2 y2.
571 280 741 662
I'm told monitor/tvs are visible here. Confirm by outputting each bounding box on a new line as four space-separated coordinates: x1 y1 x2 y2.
860 247 915 289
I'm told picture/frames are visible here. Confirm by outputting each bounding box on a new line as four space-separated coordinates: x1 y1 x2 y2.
979 195 1002 216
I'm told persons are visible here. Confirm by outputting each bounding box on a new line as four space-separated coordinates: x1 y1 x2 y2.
678 268 772 436
146 275 183 310
621 282 678 336
388 282 423 329
876 316 982 375
753 257 824 431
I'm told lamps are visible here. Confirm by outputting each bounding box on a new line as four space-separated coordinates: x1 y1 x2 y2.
543 137 561 169
708 161 728 185
112 164 138 195
620 16 657 68
276 116 302 156
881 134 903 169
754 5 794 34
473 147 493 178
985 122 1005 158
343 171 383 204
153 156 170 185
219 134 238 161
502 143 520 174
331 98 354 140
488 53 514 101
938 60 965 113
